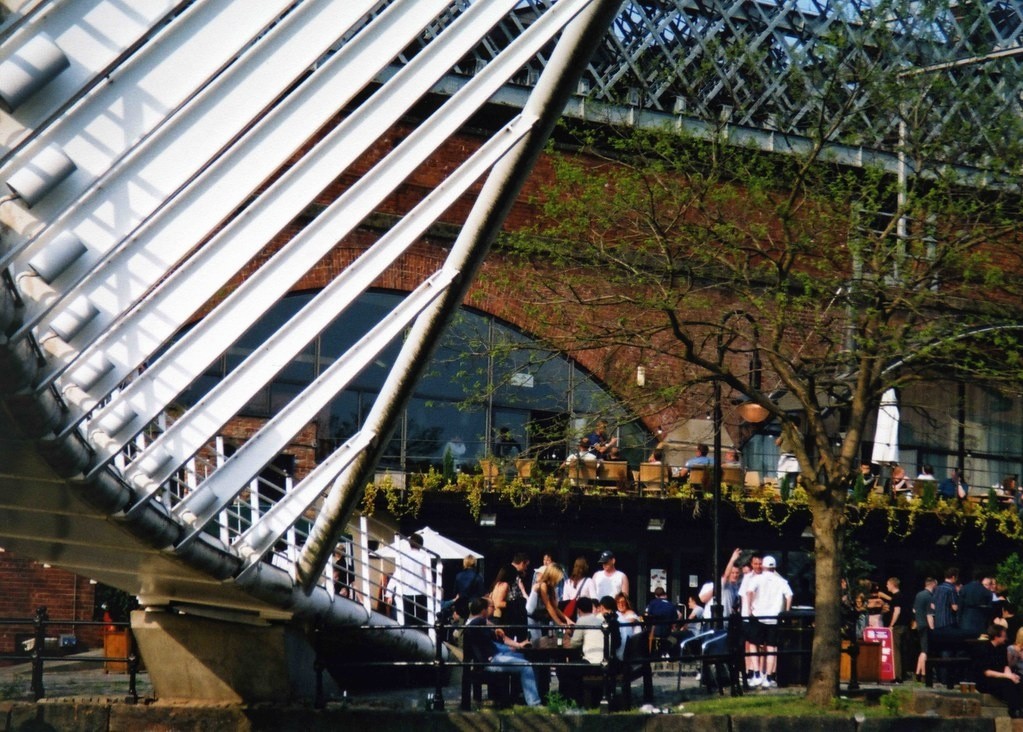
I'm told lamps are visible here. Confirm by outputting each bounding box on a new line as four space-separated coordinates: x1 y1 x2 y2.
636 365 646 386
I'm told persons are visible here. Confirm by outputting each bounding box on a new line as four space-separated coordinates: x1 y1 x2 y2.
995 479 1023 516
557 420 635 489
333 535 793 714
444 433 467 472
721 451 742 466
638 449 673 482
776 417 805 497
911 569 1023 718
679 444 714 478
840 576 908 685
891 464 969 511
493 427 525 480
849 463 878 502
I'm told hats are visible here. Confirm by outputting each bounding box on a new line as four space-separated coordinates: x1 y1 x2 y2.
597 550 617 563
761 555 777 568
654 587 667 598
533 565 548 575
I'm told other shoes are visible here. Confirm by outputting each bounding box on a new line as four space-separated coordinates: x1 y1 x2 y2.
747 670 778 689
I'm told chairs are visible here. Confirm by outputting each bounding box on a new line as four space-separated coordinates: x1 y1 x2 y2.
466 609 815 708
915 479 937 499
479 457 744 497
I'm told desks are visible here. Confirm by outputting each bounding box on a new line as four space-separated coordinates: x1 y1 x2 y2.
517 647 580 703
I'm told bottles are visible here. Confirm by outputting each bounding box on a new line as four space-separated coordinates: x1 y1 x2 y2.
526 630 530 647
557 627 564 648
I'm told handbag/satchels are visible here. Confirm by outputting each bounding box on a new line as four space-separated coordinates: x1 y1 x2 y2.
555 599 578 624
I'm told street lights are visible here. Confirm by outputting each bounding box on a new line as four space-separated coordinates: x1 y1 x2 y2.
709 308 774 629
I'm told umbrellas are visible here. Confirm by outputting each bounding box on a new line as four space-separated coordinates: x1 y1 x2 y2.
872 388 899 466
